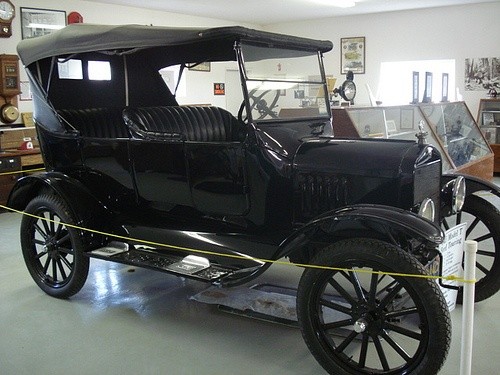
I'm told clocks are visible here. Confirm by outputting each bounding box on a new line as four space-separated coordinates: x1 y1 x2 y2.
0 0 15 38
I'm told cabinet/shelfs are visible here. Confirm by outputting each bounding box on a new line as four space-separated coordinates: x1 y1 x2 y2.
0 152 21 214
331 101 494 183
476 99 500 175
0 55 22 98
0 125 44 167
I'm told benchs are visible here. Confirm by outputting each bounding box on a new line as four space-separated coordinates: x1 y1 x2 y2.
56 106 130 138
123 106 247 141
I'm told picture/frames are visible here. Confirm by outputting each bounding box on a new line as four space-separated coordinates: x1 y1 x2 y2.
340 37 365 75
20 7 66 40
188 62 210 72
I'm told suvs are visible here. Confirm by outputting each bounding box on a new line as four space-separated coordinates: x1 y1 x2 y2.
6 23 500 375
